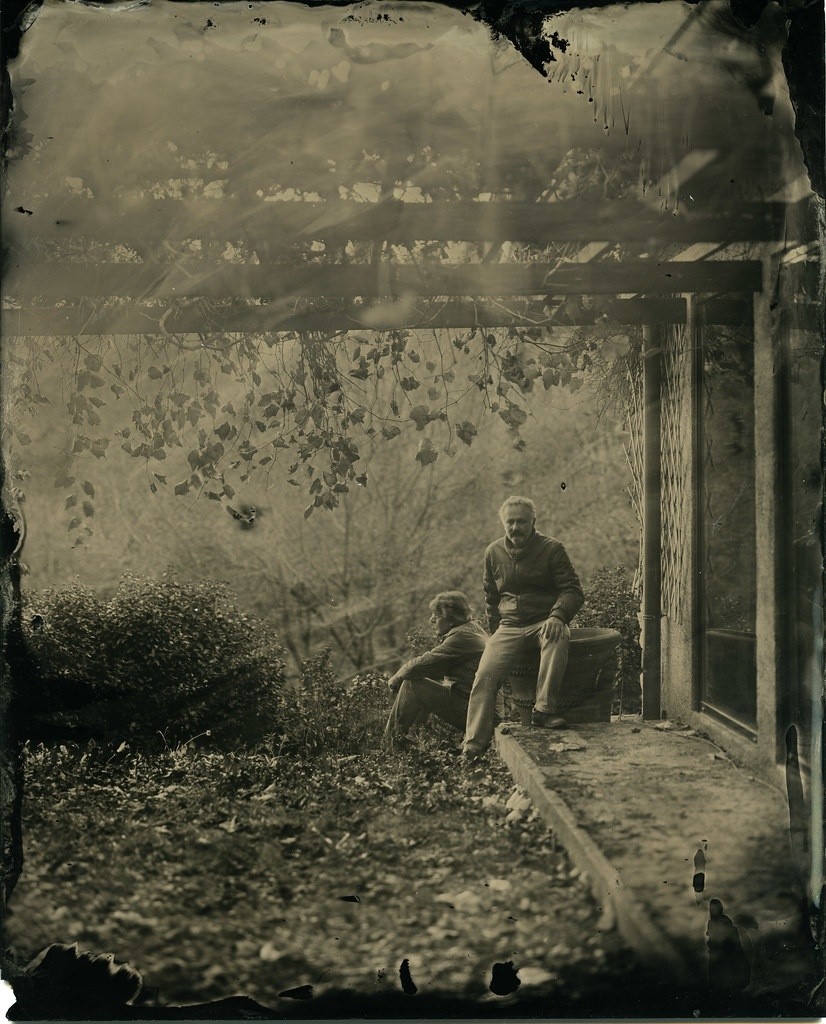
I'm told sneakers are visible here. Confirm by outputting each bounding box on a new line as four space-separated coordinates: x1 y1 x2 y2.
531 707 567 729
458 747 485 769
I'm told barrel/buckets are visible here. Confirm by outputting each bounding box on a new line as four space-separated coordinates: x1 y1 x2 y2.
705 628 756 720
511 627 623 727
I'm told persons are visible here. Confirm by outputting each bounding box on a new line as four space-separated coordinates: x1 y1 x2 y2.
462 496 585 759
783 501 826 745
381 591 504 749
